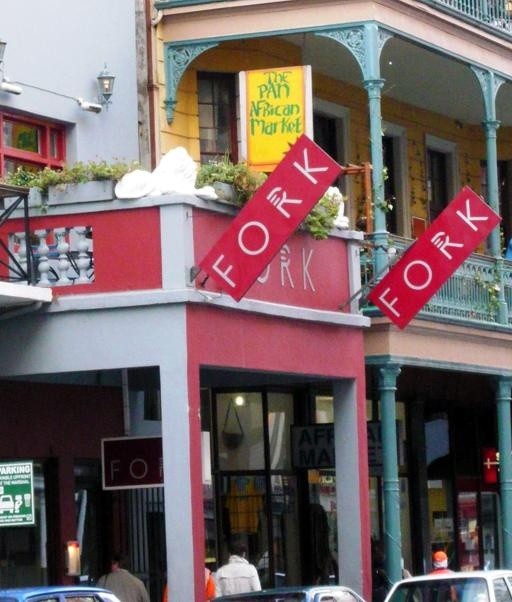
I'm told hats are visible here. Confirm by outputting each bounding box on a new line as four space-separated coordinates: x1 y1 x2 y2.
432 550 448 568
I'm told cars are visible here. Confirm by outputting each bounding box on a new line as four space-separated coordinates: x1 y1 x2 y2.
384 567 512 602
213 584 366 602
0 583 119 602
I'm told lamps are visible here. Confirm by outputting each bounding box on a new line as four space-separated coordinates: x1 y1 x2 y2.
96 61 117 114
0 40 8 79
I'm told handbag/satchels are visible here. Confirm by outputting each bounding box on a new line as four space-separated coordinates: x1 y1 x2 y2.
221 430 242 449
